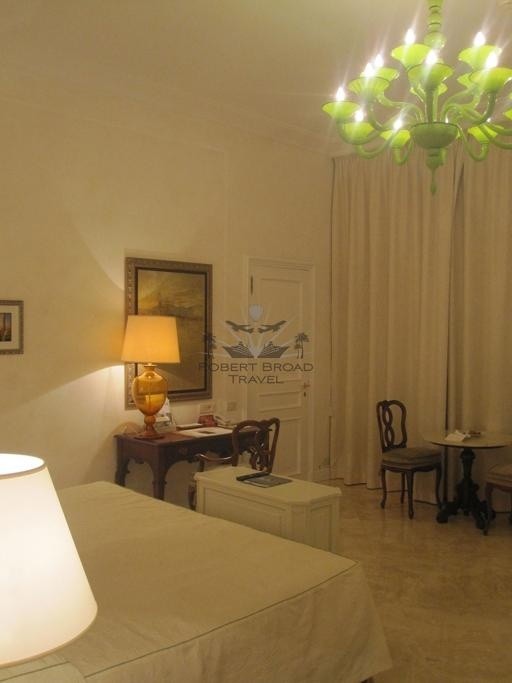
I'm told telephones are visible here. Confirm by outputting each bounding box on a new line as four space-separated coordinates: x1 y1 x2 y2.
215 414 231 426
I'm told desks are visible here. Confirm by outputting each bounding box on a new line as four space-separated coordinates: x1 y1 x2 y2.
421 429 511 531
113 421 270 512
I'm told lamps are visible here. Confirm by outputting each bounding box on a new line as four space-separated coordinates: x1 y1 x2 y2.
119 312 182 439
321 0 512 196
0 454 100 674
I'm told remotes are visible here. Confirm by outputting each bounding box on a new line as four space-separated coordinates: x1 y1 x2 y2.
237 471 268 481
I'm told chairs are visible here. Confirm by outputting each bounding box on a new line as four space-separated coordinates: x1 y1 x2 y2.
189 417 280 514
482 460 511 535
376 399 443 517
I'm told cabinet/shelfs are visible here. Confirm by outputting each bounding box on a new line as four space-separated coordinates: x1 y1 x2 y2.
193 465 342 553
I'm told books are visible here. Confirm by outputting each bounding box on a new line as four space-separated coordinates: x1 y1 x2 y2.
443 430 485 442
244 474 293 488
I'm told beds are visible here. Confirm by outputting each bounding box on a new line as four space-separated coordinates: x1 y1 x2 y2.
1 481 393 683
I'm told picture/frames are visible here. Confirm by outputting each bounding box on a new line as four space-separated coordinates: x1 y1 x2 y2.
123 255 214 411
1 299 25 354
